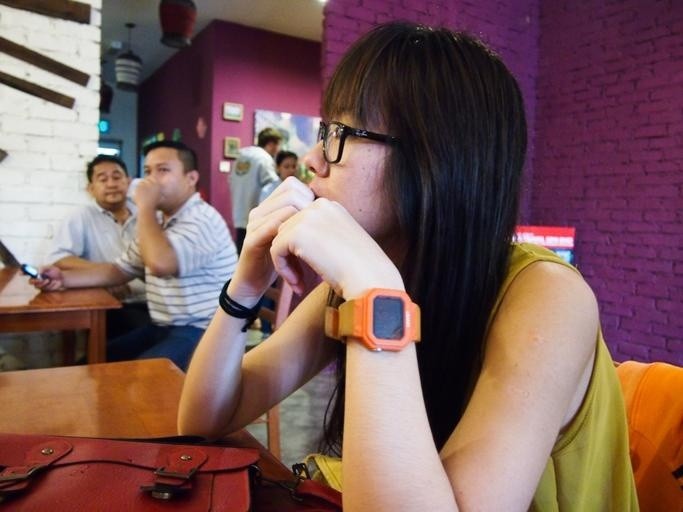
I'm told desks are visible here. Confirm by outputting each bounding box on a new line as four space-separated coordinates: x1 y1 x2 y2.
0 265 125 364
0 356 302 511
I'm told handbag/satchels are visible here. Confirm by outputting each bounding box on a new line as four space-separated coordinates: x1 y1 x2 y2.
0 432 264 512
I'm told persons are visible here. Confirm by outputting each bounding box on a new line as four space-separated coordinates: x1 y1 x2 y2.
228 128 283 259
256 151 299 205
41 154 167 358
178 21 640 512
29 140 241 373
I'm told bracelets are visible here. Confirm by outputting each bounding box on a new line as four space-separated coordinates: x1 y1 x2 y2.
218 277 263 332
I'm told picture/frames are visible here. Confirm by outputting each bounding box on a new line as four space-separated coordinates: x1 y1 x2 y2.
222 102 243 122
224 137 240 158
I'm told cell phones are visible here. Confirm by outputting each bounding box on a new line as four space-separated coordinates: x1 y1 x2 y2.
20 264 52 284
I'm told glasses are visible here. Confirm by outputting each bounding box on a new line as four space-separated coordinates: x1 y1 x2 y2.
317 121 397 164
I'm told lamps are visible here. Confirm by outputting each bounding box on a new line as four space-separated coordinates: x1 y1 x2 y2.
157 0 198 48
113 23 142 90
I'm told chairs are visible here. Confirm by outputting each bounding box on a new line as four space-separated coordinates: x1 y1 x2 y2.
242 271 297 460
613 361 682 512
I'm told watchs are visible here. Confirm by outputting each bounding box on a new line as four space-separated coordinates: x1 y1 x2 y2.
325 287 423 350
